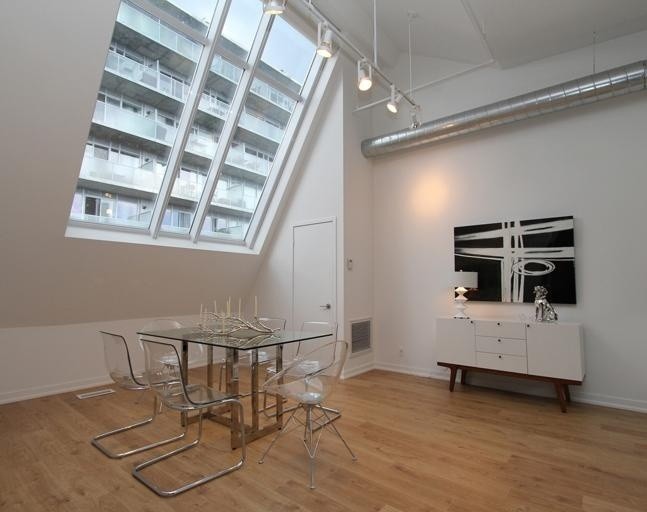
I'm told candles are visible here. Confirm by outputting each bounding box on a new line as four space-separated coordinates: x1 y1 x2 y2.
200 295 258 317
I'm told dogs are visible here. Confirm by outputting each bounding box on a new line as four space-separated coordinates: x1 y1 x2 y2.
534 286 558 322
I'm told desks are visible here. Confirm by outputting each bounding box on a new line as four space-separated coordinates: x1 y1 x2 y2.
134 324 335 451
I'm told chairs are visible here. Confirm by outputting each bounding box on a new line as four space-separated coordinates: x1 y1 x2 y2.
132 338 248 499
90 312 358 499
198 314 357 490
89 331 188 460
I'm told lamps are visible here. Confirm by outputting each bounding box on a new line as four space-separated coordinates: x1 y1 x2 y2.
262 0 422 131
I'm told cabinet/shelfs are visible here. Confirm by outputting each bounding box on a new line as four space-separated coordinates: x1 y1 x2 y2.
435 315 585 412
